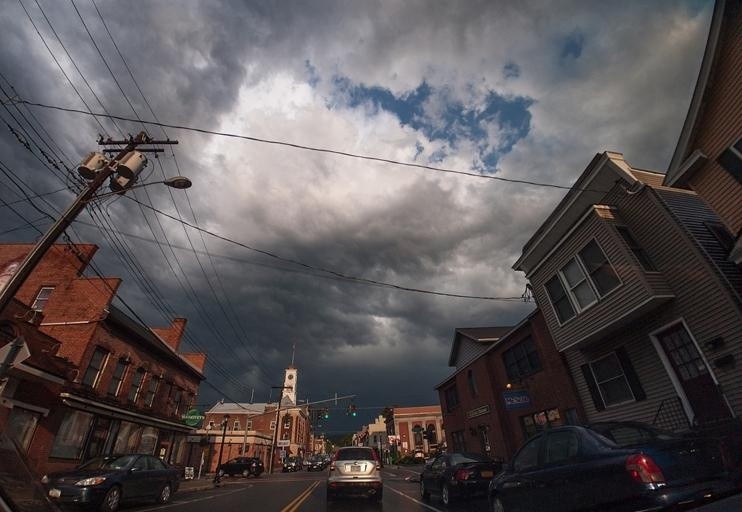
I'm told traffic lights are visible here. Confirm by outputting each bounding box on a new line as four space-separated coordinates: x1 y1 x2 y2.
349 402 358 417
317 411 329 419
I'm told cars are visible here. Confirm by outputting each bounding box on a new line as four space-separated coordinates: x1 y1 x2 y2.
33 454 181 512
215 456 264 478
283 454 331 472
327 446 382 504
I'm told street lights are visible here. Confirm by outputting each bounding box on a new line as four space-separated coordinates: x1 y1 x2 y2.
212 413 231 483
0 176 192 314
